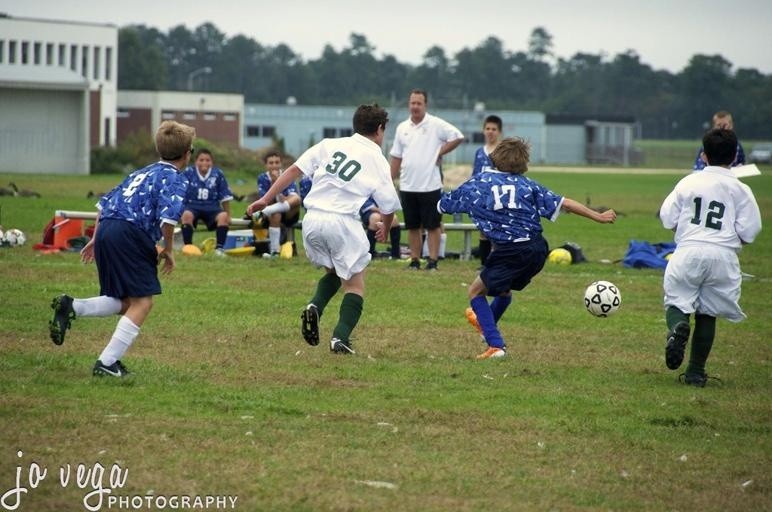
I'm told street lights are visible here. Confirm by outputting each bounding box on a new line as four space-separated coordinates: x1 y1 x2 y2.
187 64 213 90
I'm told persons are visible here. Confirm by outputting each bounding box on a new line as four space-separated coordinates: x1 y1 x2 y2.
658 127 762 386
694 110 745 172
471 115 504 272
246 102 403 353
181 88 464 270
438 134 618 358
50 118 196 379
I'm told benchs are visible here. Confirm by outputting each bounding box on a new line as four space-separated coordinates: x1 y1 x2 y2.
53 211 482 264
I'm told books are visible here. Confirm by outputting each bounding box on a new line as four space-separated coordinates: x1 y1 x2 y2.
731 163 761 179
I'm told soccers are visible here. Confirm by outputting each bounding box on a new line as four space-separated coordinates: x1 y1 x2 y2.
584 281 622 318
548 248 572 267
0 228 27 248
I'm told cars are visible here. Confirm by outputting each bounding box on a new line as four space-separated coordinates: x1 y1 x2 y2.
748 140 772 165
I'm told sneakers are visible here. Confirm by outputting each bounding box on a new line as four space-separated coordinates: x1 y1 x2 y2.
301 303 320 345
408 260 438 272
665 321 690 370
49 294 76 344
93 359 130 377
686 372 707 388
477 347 506 360
466 307 485 340
330 337 354 354
